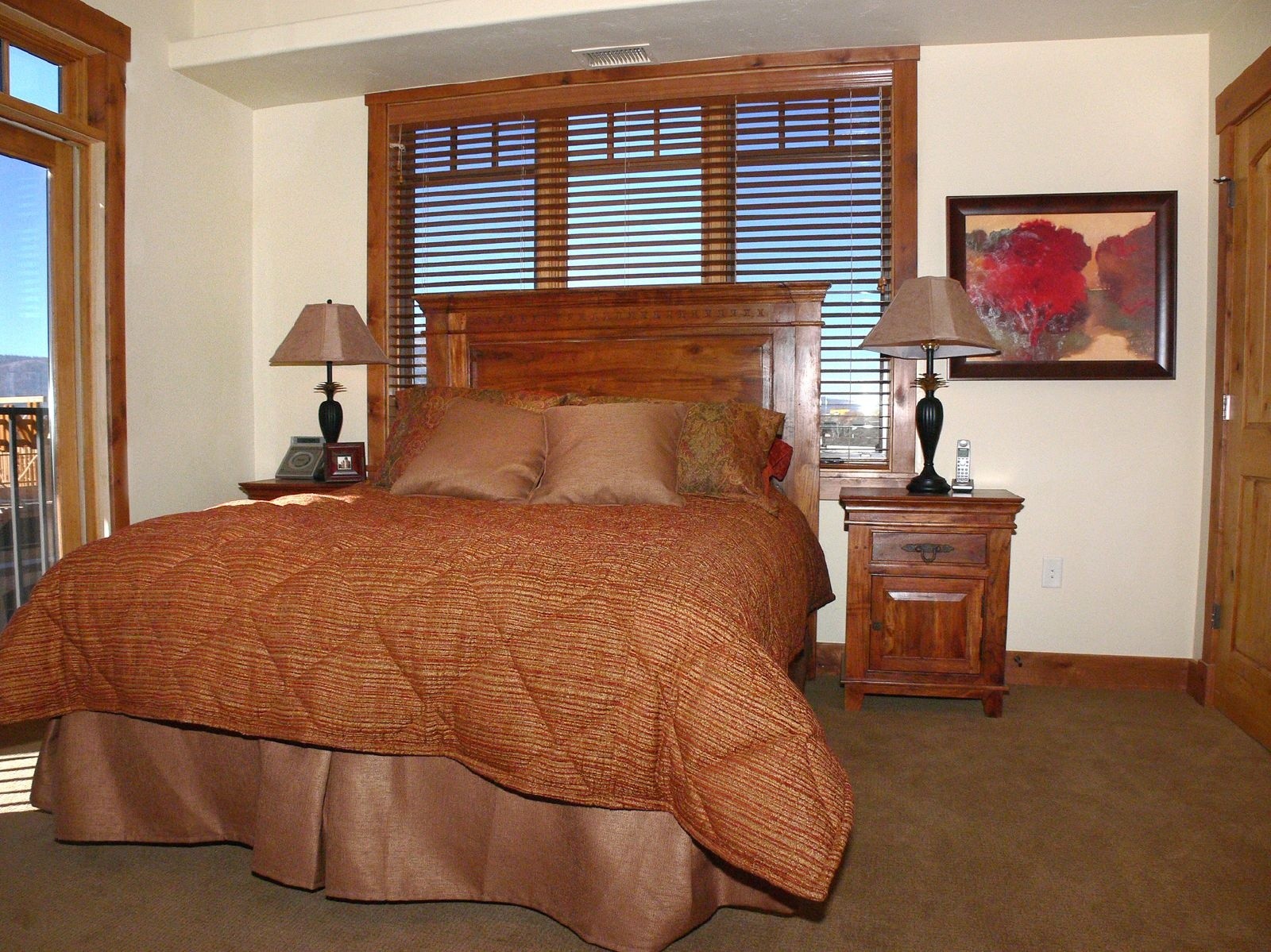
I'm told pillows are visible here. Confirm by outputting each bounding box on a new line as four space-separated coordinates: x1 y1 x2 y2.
564 391 788 519
371 387 577 489
527 405 690 510
390 395 547 507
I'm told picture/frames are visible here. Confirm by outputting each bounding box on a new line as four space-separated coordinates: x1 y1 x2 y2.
945 191 1176 381
323 442 367 483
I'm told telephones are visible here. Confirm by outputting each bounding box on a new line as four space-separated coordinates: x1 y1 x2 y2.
951 439 974 494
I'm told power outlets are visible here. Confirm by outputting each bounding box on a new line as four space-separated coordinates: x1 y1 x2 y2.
1041 556 1064 589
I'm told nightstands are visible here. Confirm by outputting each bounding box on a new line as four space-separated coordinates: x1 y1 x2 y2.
838 485 1027 719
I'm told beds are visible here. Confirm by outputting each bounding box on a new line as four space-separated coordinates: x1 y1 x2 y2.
0 280 861 952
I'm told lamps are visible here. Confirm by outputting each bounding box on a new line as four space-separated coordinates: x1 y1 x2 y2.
268 298 390 444
856 275 1002 495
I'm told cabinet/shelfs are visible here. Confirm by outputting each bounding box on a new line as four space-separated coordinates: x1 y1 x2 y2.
238 480 357 501
